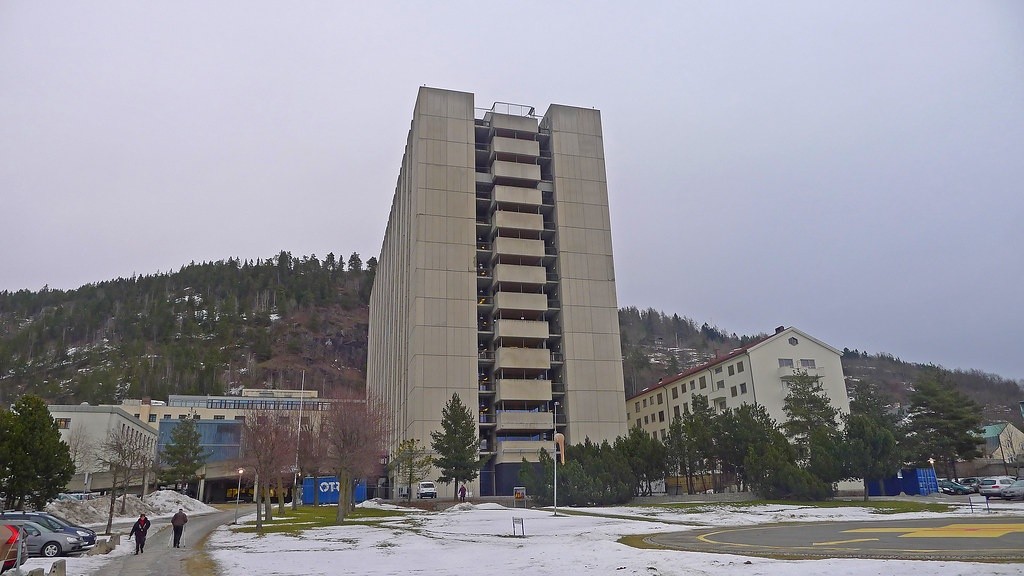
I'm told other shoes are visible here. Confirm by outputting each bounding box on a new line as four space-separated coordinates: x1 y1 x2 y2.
134 552 138 555
141 548 143 553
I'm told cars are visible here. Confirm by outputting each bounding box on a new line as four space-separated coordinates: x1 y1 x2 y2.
0 519 84 557
937 476 986 495
1001 480 1024 501
161 483 188 491
53 493 76 502
0 525 29 575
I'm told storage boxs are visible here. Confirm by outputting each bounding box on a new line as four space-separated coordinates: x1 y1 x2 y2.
862 466 940 497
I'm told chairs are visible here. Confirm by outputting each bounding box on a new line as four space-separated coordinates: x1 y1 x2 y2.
479 439 488 450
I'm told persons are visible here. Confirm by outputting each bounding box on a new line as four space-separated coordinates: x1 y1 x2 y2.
459 485 467 502
130 514 150 555
171 509 188 548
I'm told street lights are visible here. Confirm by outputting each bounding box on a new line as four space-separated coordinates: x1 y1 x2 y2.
234 468 245 524
291 370 306 509
554 401 560 515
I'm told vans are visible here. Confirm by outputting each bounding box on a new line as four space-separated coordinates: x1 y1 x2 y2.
416 482 437 498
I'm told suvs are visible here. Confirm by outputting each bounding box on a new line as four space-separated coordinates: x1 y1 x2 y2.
0 509 98 549
978 476 1015 498
